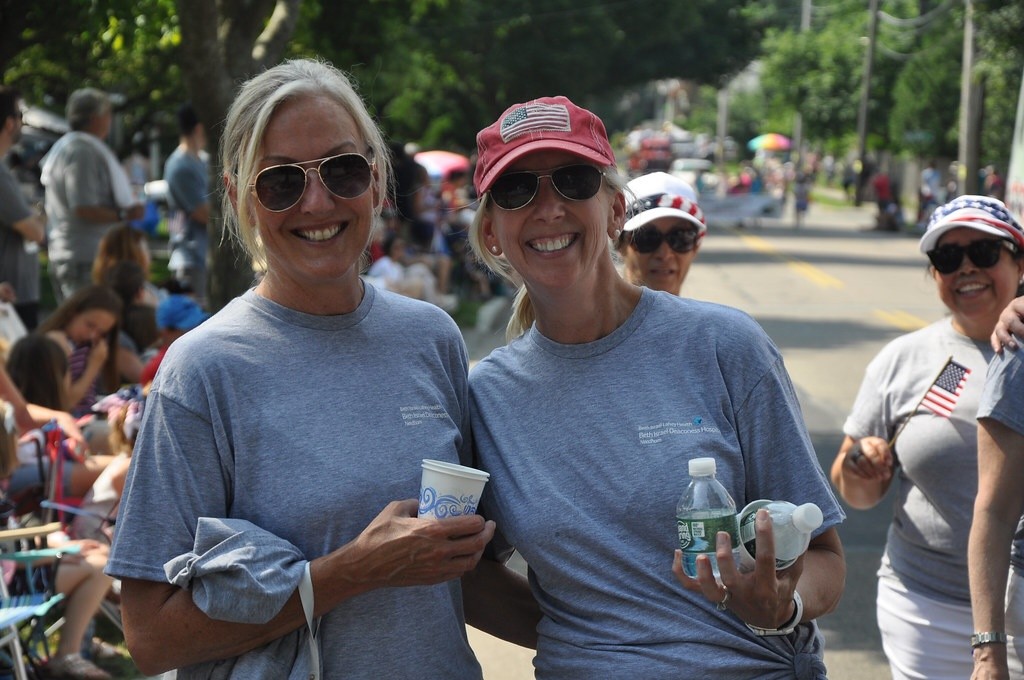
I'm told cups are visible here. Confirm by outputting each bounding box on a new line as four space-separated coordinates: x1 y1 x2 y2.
422 458 492 479
736 498 799 576
416 464 489 520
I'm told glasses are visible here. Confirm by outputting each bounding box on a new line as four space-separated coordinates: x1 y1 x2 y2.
233 153 377 213
6 112 23 120
489 163 606 211
628 223 698 253
927 240 1017 274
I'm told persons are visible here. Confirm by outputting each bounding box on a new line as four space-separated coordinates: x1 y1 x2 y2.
829 195 1024 680
610 169 709 298
729 149 1003 234
106 59 541 680
464 95 846 680
0 80 515 680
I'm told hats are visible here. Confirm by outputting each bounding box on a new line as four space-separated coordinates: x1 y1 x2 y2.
623 171 706 232
920 195 1024 252
474 95 617 198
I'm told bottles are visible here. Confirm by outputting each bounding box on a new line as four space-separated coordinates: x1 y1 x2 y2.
740 500 824 568
676 457 742 578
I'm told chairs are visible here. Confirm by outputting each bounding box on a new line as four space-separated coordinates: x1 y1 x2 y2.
0 301 124 680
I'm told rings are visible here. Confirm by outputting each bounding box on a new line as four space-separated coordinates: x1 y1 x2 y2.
721 592 728 604
850 451 861 462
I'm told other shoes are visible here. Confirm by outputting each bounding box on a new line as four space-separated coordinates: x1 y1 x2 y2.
98 598 126 640
82 637 124 668
48 658 114 680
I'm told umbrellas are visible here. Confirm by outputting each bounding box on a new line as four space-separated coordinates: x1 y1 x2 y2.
748 133 792 151
414 149 469 177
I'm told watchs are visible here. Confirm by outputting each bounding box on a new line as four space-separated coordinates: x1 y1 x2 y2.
971 633 1007 647
744 590 803 637
